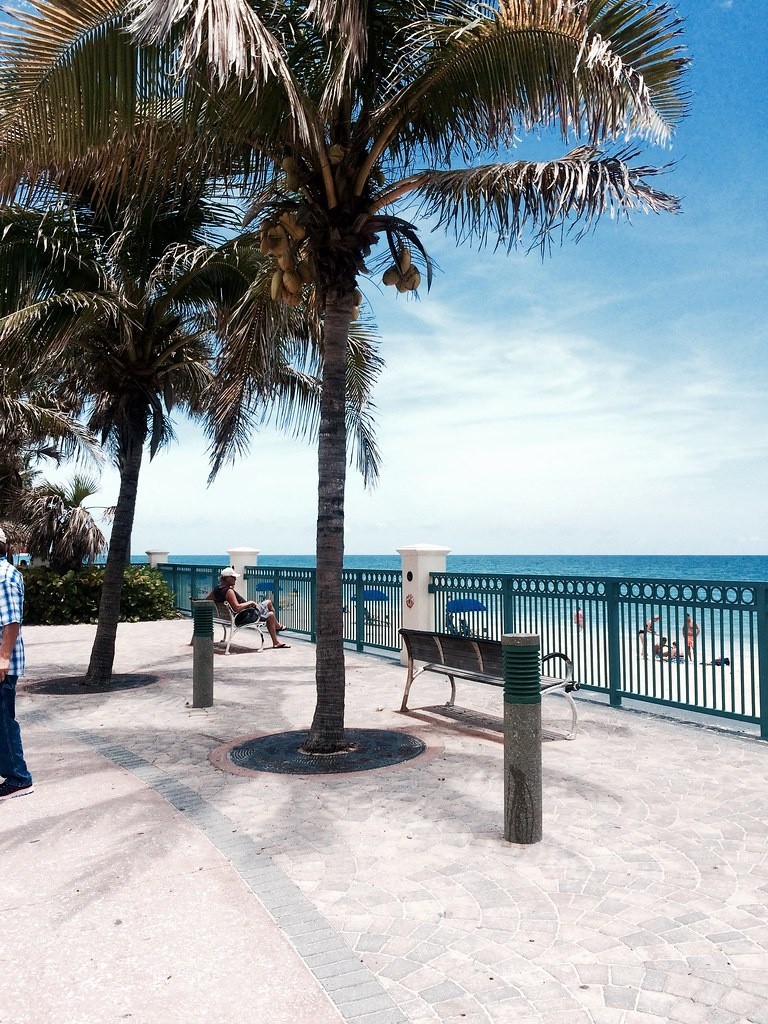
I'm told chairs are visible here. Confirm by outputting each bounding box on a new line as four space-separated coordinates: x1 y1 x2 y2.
447 615 465 636
459 618 489 640
363 608 391 627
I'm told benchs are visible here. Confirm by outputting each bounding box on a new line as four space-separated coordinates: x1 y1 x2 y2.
398 627 582 740
187 596 267 655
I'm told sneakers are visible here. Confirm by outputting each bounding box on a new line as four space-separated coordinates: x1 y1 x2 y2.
0 781 34 800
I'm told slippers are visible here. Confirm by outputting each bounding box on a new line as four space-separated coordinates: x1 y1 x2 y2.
276 625 287 631
274 644 291 648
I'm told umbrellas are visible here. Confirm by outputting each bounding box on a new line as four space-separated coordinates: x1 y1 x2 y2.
256 582 284 591
186 573 208 581
350 589 389 602
446 598 488 612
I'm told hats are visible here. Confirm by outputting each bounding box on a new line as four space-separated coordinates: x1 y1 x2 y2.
221 568 240 577
0 528 7 543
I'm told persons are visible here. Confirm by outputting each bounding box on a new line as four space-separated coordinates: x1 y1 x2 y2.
205 567 291 648
0 526 35 801
258 586 300 611
573 605 585 637
444 595 485 640
342 604 369 625
19 559 27 567
638 612 730 666
